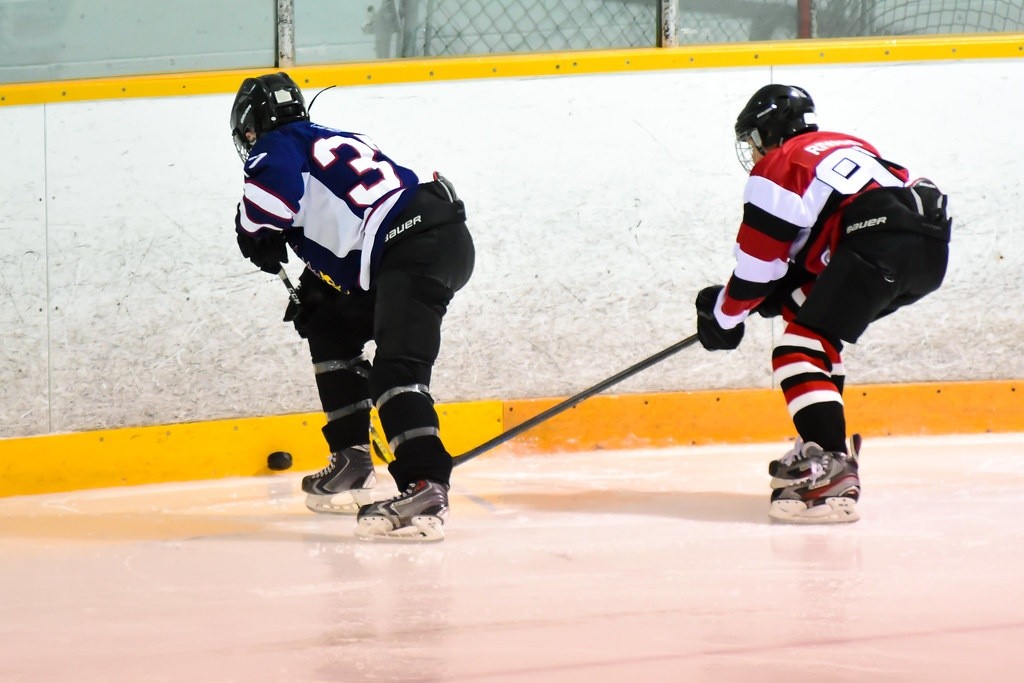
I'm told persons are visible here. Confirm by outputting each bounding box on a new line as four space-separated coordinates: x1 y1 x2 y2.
230 72 475 543
696 84 953 524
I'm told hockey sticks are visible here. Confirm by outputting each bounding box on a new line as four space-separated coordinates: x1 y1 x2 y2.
277 266 394 464
449 334 700 466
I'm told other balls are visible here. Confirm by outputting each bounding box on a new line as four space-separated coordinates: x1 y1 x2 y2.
267 450 293 471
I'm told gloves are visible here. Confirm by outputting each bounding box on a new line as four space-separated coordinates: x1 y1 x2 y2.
758 284 782 318
696 285 744 351
235 235 289 275
283 266 342 338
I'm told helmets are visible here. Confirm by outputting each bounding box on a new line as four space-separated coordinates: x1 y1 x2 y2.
230 72 310 163
735 84 819 175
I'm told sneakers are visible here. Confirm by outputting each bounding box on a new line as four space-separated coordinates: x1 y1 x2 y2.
302 447 377 513
768 433 862 524
769 434 814 489
353 478 450 544
